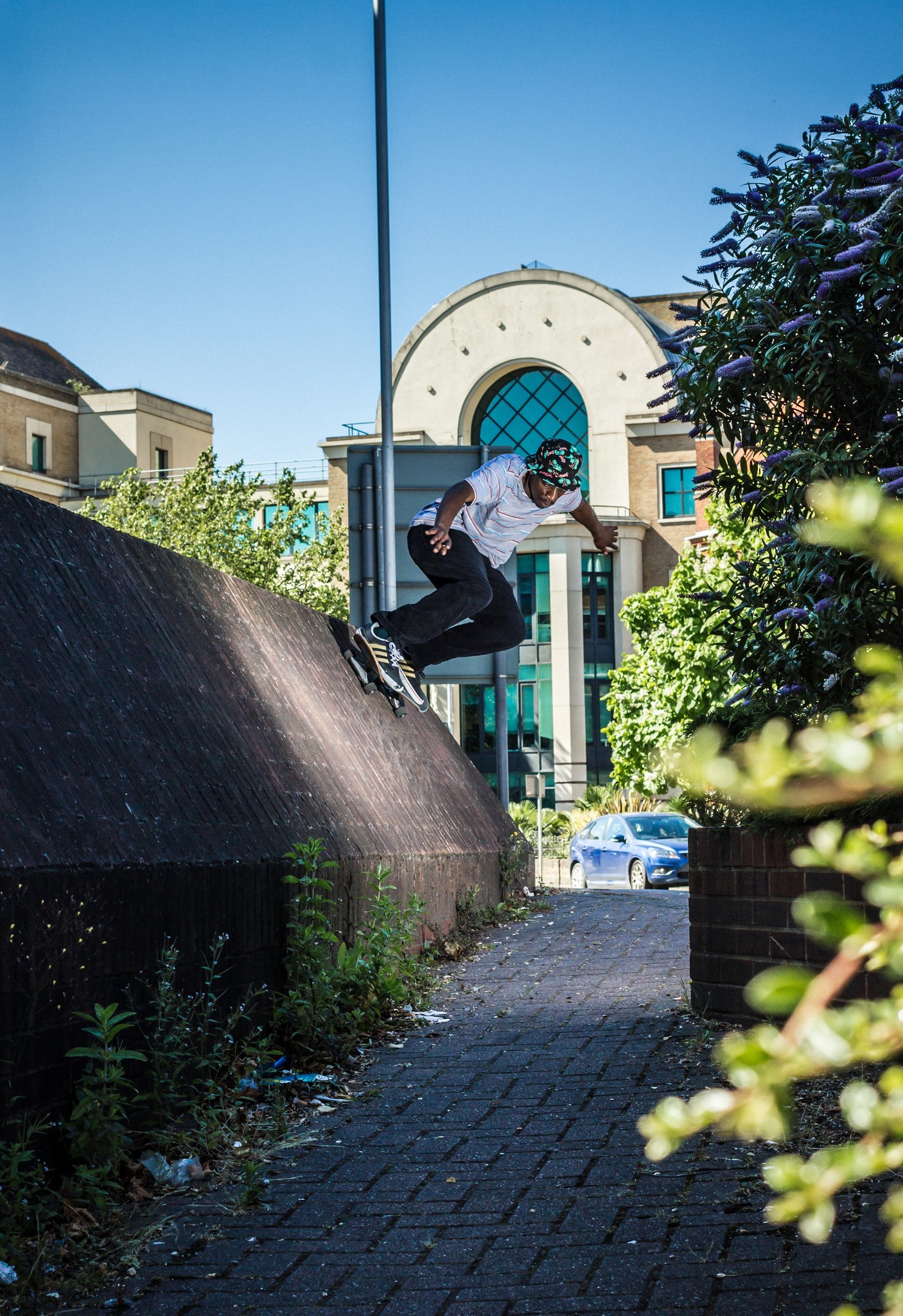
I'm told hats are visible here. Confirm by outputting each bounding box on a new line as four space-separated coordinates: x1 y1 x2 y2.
524 438 583 492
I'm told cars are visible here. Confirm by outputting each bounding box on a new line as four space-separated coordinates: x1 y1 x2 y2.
568 812 703 890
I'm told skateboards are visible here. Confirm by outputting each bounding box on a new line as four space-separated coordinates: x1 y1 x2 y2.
334 616 432 716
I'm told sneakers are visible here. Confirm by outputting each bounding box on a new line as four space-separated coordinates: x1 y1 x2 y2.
356 622 404 693
397 648 424 705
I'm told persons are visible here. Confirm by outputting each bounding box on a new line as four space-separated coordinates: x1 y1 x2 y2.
346 437 622 714
